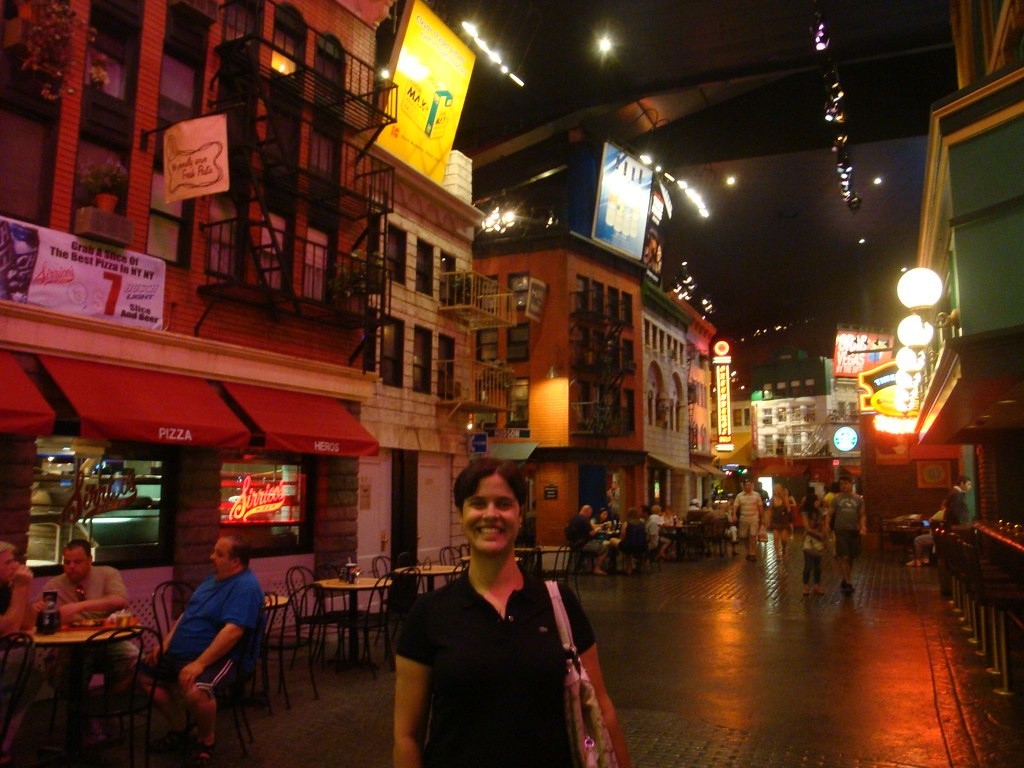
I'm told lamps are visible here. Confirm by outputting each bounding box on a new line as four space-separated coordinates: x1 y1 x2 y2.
811 7 862 213
893 267 959 413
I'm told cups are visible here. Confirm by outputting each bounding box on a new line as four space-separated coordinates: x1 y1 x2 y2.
116 611 131 630
346 564 357 584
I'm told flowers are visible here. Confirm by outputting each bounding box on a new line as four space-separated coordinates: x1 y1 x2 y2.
90 52 111 84
79 155 130 194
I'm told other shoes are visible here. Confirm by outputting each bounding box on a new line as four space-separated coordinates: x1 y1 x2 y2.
82 730 109 746
841 579 854 594
594 569 633 577
746 554 756 562
789 535 794 540
151 725 219 765
775 554 787 562
802 586 819 596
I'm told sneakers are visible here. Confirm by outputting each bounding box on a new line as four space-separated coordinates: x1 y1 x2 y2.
907 557 930 568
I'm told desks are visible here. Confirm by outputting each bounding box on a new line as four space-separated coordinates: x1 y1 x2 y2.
599 531 621 576
514 545 572 585
394 566 465 592
312 578 394 672
461 555 523 570
8 622 145 768
660 526 698 561
264 594 290 609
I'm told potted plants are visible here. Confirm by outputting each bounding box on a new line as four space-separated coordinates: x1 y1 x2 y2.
324 246 393 300
659 405 669 420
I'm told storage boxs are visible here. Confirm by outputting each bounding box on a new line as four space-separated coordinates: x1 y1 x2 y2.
444 149 472 204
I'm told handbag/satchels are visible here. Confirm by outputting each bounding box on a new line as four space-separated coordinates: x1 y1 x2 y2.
758 525 769 543
546 579 623 768
803 534 826 558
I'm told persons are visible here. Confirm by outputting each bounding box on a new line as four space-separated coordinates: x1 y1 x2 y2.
0 539 130 768
566 472 972 596
133 530 266 768
391 455 633 768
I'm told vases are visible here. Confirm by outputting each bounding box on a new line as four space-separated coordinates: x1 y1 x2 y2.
96 194 118 213
93 83 103 91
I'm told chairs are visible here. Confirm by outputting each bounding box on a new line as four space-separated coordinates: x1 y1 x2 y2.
0 508 740 768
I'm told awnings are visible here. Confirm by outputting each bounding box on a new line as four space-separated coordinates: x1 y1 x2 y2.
1 351 378 456
